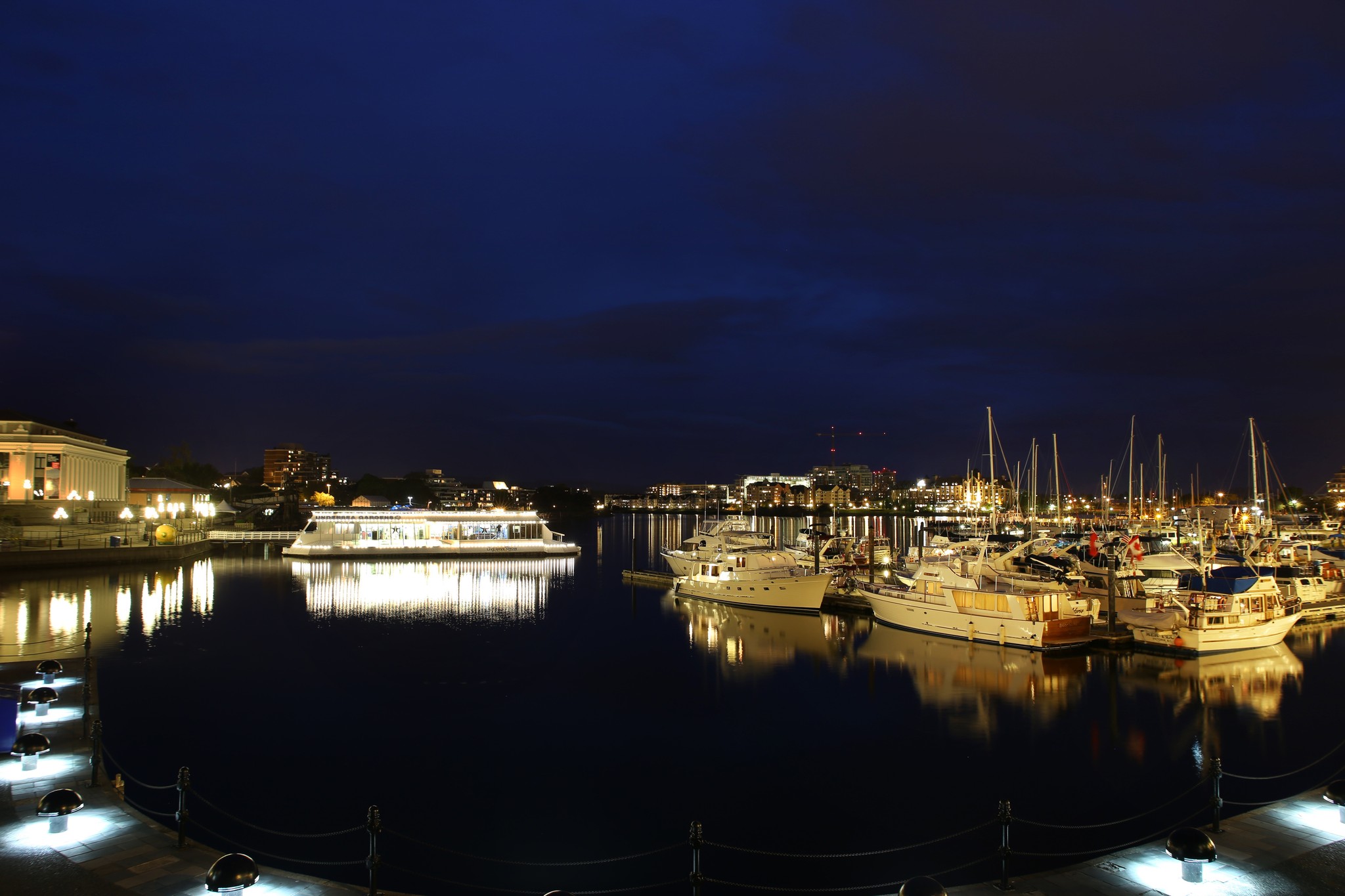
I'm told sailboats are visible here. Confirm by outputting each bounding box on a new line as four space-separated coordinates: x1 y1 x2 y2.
924 407 1345 618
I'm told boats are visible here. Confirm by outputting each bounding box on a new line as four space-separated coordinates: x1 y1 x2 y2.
658 512 1096 649
1117 588 1307 651
281 510 581 558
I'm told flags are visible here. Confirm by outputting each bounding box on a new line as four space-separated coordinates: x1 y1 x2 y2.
842 553 846 564
1227 529 1236 547
1247 528 1256 549
1157 596 1166 613
976 553 979 560
1128 534 1143 561
1047 546 1053 553
1088 528 1099 557
1077 583 1082 598
1121 533 1131 544
1043 540 1049 547
1050 552 1059 559
860 545 864 555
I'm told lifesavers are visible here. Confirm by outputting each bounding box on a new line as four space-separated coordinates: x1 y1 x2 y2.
1219 598 1227 610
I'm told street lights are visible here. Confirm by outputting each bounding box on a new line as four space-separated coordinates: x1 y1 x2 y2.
3 478 214 549
